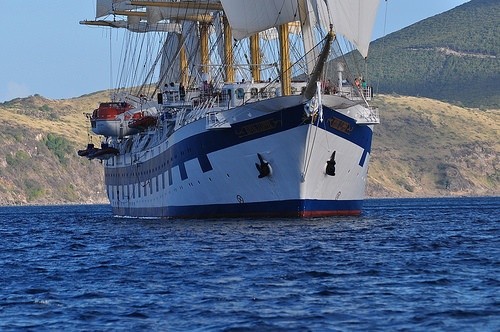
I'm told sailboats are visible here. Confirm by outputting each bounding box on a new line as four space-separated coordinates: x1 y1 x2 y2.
75 0 383 218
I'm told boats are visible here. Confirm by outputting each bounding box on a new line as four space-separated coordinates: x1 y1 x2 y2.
89 102 143 138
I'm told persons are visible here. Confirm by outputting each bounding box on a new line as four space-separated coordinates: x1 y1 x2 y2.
355 78 361 90
362 80 367 89
